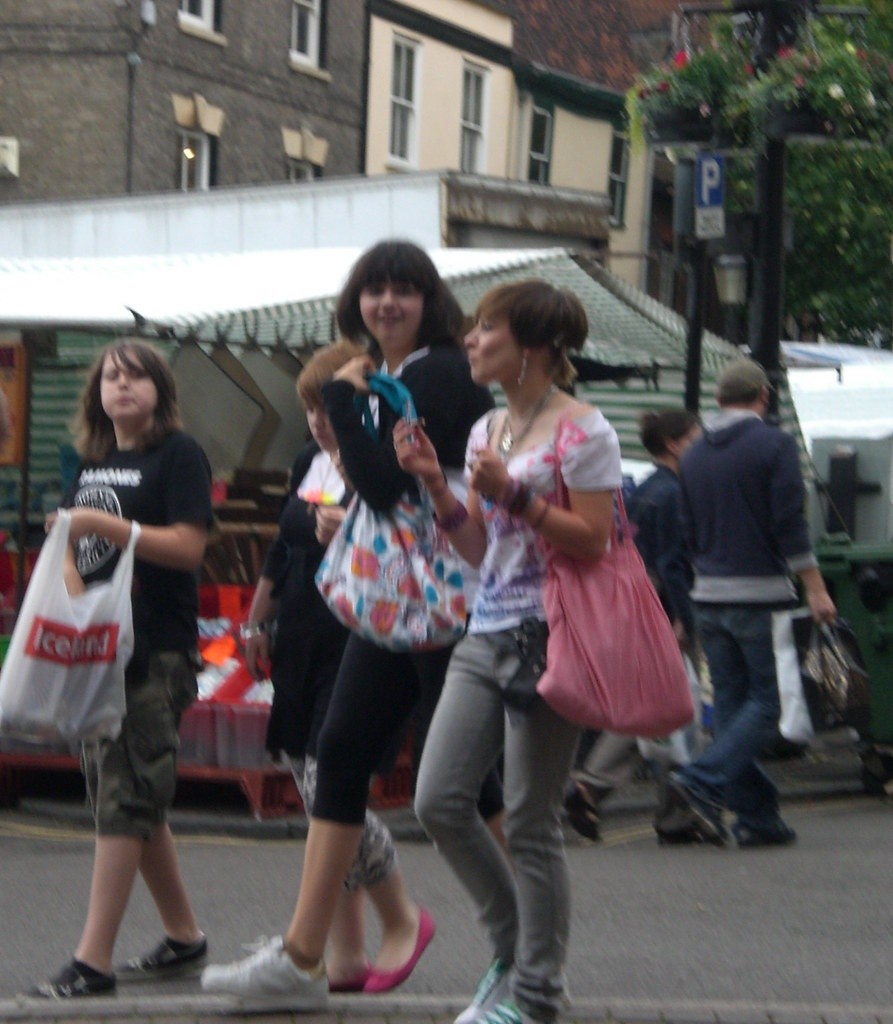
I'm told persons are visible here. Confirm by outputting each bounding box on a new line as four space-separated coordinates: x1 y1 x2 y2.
391 278 623 1023
668 357 837 847
14 337 208 1001
570 408 712 838
243 341 434 998
199 241 509 1012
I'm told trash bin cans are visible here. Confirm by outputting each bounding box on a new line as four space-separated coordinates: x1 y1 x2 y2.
814 542 892 794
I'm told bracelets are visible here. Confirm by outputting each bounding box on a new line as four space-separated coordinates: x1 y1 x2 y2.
239 624 263 639
502 479 534 516
530 502 550 531
432 498 466 532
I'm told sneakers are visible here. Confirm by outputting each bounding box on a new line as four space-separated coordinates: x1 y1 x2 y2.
454 959 541 1024
201 932 328 1002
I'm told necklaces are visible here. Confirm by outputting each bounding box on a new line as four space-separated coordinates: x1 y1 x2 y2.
499 384 558 462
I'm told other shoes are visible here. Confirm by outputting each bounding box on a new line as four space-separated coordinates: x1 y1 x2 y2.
118 934 209 982
364 909 435 993
330 963 372 992
736 822 795 845
17 963 116 998
663 772 732 848
565 780 599 840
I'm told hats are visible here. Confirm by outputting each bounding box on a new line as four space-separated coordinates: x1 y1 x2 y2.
716 360 776 398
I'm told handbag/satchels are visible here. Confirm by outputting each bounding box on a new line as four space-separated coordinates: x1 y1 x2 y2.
315 374 465 652
0 508 141 743
535 408 693 736
770 609 877 746
639 650 710 767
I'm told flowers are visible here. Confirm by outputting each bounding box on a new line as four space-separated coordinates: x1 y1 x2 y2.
763 28 870 108
636 44 750 122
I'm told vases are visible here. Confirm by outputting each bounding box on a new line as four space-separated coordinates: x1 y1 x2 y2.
644 102 744 141
768 88 876 148
